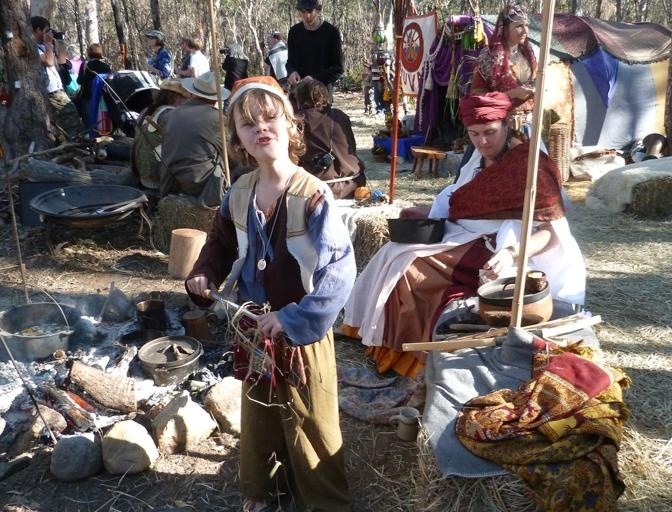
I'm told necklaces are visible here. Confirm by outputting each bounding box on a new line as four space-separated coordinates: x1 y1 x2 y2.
510 50 522 65
253 172 292 271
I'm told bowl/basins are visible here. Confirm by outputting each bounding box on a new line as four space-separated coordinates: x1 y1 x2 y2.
386 218 445 244
26 184 148 228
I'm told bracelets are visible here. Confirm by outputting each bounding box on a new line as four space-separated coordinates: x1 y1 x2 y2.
505 245 522 263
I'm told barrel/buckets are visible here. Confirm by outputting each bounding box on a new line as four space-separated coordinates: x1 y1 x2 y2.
138 335 204 387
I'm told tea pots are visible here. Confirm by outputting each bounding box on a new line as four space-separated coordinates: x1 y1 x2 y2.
177 309 219 343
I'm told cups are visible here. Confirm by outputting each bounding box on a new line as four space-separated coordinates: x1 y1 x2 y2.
524 270 547 294
389 407 420 442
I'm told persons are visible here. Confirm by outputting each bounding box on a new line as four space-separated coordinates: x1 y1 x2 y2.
184 76 358 512
30 2 367 211
339 90 588 383
452 2 575 214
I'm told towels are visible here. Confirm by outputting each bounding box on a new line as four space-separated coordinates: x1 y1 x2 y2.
420 327 534 478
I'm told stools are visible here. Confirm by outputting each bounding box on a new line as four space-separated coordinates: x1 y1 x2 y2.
411 146 447 178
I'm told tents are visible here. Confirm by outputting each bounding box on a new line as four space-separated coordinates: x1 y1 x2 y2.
416 14 671 162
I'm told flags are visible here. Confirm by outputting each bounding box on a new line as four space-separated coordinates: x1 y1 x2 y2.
398 9 439 96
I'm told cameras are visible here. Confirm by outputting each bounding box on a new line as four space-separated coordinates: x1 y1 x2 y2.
220 47 230 55
48 28 65 40
314 153 335 172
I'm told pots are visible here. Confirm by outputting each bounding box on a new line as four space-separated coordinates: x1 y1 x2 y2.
476 276 553 328
136 333 208 390
131 281 167 341
0 301 82 362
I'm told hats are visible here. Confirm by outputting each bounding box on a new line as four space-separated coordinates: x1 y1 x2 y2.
160 79 192 99
181 71 231 102
145 30 166 42
458 90 514 128
226 73 294 122
268 30 282 41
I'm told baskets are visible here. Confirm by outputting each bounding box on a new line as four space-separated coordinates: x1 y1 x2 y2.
547 120 571 183
